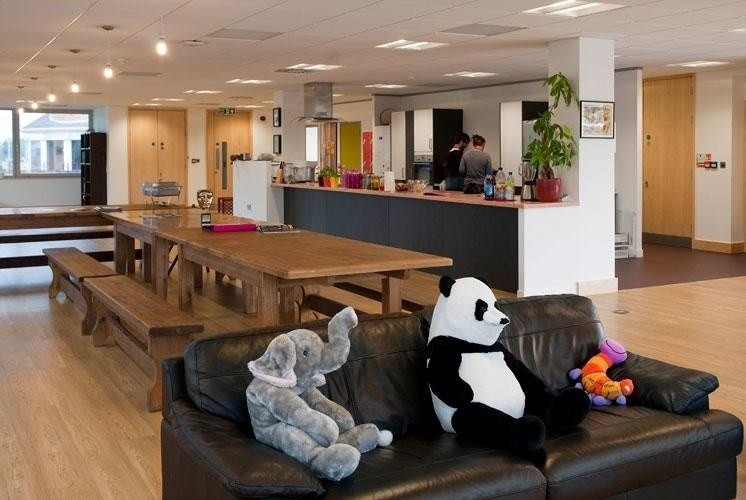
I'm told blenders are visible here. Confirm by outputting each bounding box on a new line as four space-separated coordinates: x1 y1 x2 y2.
518 159 539 202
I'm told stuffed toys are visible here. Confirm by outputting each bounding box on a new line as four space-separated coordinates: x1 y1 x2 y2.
427 274 591 453
245 306 381 481
568 337 634 408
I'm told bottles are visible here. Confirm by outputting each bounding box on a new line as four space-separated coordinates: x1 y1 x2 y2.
506 171 515 200
492 170 498 186
523 178 532 200
494 167 505 201
483 171 494 200
342 171 363 189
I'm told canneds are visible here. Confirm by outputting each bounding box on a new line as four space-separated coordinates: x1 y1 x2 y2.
367 175 372 189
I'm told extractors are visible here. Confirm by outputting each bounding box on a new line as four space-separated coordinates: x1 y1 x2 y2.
298 82 344 127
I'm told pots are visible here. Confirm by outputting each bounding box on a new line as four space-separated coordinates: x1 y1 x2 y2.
290 166 316 182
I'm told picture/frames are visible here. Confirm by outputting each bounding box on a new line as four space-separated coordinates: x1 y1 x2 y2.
273 108 282 127
580 100 615 139
272 134 281 154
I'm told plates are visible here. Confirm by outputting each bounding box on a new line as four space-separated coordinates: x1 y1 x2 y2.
408 179 430 193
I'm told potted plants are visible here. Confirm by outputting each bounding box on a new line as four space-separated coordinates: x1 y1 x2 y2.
322 167 330 186
316 168 323 186
520 71 579 202
330 168 340 188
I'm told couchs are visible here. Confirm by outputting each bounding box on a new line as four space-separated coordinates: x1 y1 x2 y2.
160 294 744 500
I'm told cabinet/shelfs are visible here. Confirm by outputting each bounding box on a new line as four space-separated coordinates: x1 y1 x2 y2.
80 132 107 205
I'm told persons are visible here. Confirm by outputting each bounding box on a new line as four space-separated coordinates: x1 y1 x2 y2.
459 135 493 192
445 132 471 191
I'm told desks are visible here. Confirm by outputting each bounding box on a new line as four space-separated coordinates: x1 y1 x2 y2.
0 204 196 269
154 225 453 328
100 207 265 300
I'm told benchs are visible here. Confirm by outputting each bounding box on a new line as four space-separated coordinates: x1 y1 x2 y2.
42 245 118 337
82 274 196 411
351 270 516 308
